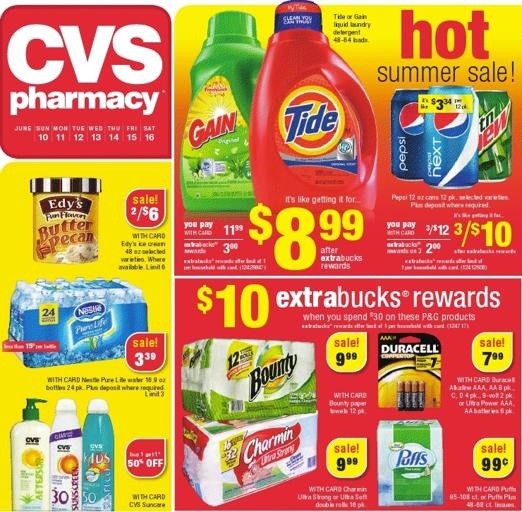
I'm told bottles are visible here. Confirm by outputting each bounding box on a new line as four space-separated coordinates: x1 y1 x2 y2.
7 278 145 362
49 398 115 511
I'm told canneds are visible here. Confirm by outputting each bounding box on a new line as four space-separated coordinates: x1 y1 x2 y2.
424 85 479 189
391 89 429 182
476 90 512 183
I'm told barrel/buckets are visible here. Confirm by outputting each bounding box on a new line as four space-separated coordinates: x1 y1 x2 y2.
248 0 379 211
180 10 266 213
